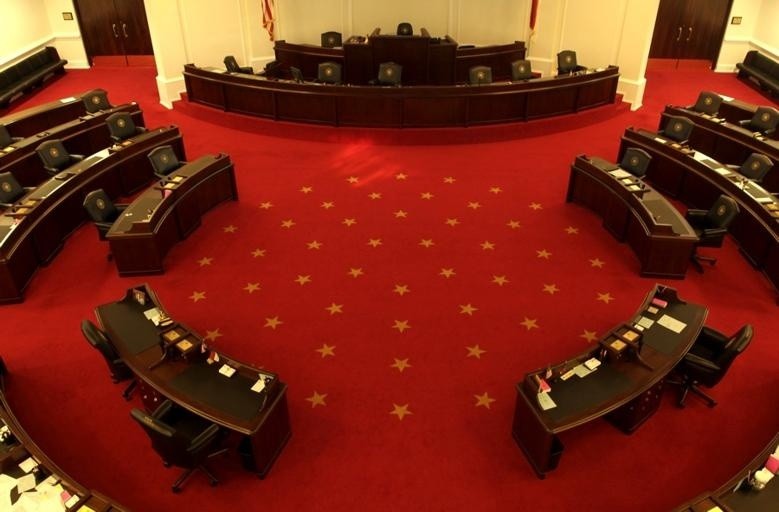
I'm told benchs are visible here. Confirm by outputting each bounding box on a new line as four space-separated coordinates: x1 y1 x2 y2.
1 46 68 108
736 50 779 104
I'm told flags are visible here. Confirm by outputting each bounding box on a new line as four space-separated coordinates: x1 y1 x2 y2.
261 1 274 41
531 0 538 30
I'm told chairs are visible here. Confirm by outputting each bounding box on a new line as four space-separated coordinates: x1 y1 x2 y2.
667 326 754 408
615 90 779 276
0 88 197 262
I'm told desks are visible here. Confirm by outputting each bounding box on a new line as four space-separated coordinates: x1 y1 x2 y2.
1 127 187 305
80 283 296 493
181 62 620 130
105 145 237 276
273 39 526 83
564 149 702 280
512 285 709 480
